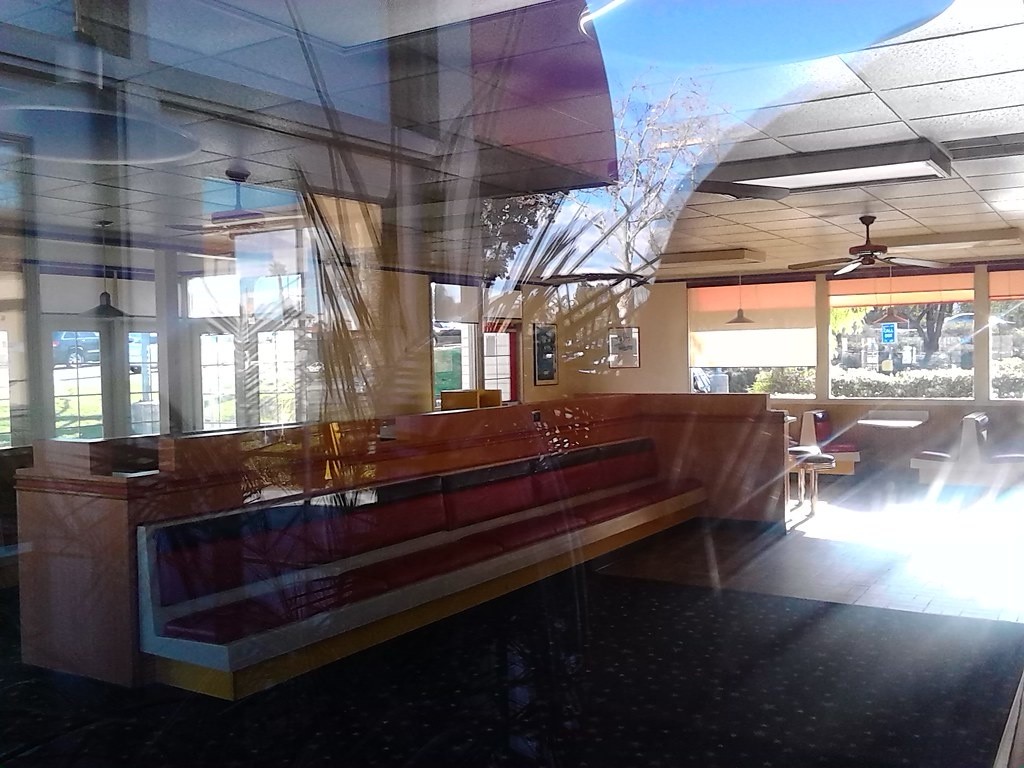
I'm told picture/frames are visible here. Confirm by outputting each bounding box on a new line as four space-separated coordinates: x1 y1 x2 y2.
608 327 640 369
533 323 559 385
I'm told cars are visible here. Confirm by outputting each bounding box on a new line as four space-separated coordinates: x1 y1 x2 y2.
52 331 101 369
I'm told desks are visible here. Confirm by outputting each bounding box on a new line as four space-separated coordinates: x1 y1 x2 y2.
856 410 930 430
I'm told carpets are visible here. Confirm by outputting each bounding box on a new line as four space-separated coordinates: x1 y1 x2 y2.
0 565 1024 768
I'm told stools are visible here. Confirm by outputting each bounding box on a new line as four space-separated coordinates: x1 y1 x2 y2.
789 446 823 503
795 454 835 515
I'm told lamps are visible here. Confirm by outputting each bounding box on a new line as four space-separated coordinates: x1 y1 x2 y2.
725 273 754 324
607 157 644 184
574 0 954 67
0 1 202 166
211 170 264 223
77 220 135 319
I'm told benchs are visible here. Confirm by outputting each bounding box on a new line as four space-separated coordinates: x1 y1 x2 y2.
789 410 863 475
135 433 714 700
910 412 1024 488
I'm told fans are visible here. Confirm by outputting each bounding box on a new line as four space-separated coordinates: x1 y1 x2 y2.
789 216 952 276
